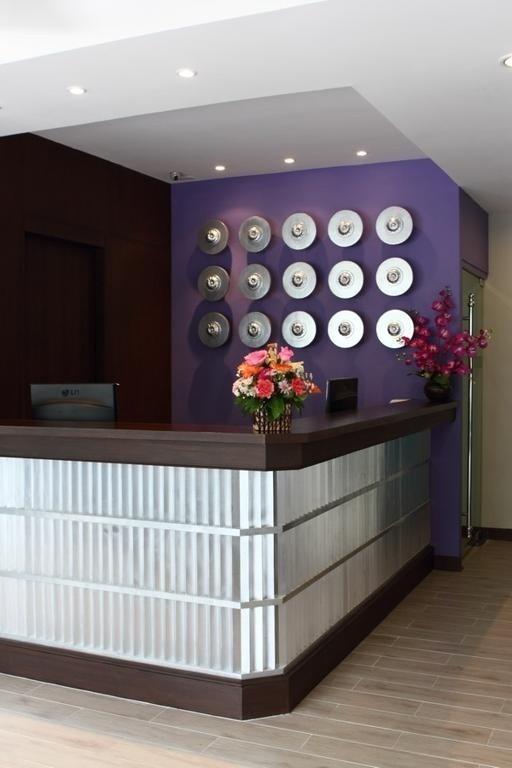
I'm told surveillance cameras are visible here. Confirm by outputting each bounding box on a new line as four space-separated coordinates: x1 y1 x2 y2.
170 172 181 181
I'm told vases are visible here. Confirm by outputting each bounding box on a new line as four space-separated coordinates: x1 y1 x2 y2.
250 397 293 436
425 372 450 404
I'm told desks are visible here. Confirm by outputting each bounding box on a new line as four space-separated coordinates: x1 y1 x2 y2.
1 396 453 721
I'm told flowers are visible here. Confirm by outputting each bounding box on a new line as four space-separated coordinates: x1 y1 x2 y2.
396 289 490 376
233 345 318 401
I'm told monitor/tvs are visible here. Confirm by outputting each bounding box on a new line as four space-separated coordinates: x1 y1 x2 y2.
323 376 359 412
29 382 120 423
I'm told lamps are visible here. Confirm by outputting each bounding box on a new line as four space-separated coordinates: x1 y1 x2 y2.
357 149 367 157
285 157 295 165
71 87 89 98
502 53 512 69
215 165 227 173
180 68 195 84
